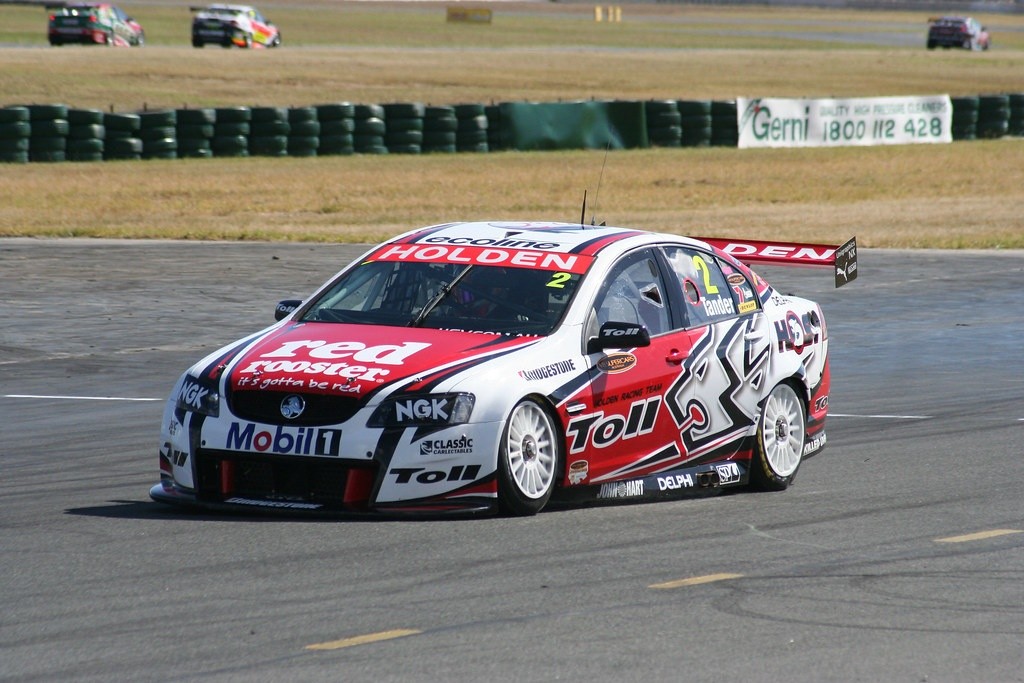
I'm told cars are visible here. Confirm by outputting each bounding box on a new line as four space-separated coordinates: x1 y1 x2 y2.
48 3 146 48
192 4 281 49
148 221 859 518
927 16 991 52
